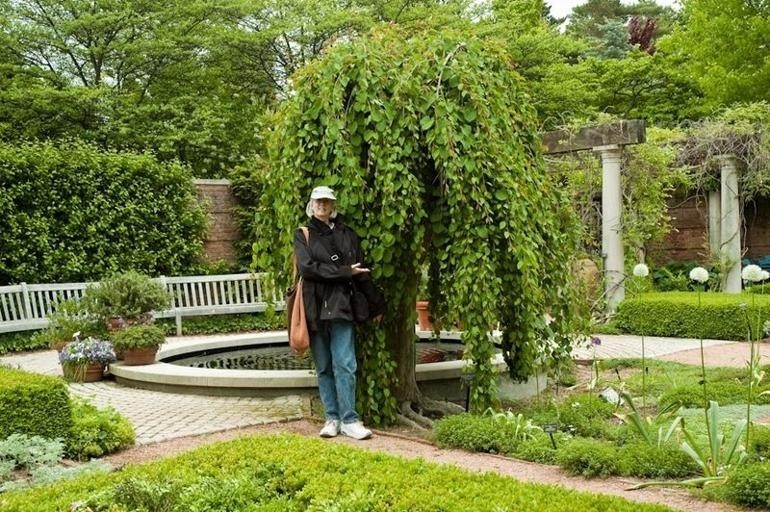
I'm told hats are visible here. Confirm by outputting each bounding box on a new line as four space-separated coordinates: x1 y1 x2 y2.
311 186 336 200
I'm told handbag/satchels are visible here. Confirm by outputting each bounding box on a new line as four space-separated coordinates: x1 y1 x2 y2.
351 272 384 322
286 284 310 354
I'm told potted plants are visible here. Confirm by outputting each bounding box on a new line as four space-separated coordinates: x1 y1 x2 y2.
42 268 173 382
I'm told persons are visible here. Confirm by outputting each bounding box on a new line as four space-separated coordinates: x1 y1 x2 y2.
295 185 385 441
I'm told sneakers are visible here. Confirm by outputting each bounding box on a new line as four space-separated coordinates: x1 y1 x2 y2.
320 419 372 440
